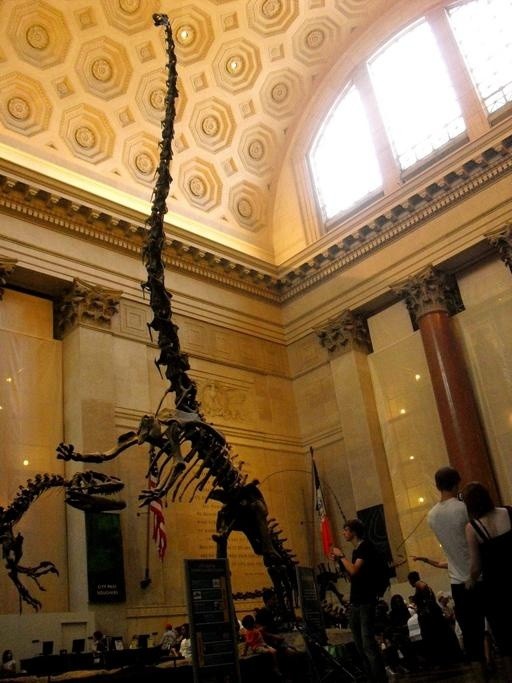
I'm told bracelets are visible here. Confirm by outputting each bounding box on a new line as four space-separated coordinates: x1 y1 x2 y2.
338 553 345 558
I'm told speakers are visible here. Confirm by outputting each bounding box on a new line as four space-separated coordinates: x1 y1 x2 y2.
356 504 397 577
85 511 125 604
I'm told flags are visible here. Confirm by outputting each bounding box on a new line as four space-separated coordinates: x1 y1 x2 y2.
148 446 166 561
311 460 335 560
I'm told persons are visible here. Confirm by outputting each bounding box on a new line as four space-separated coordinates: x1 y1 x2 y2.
158 623 191 658
333 520 388 682
236 589 284 677
2 649 17 678
89 630 107 663
376 467 512 683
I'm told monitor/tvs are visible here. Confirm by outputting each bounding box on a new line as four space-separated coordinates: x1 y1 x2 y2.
72 640 85 653
101 635 147 651
43 641 53 655
